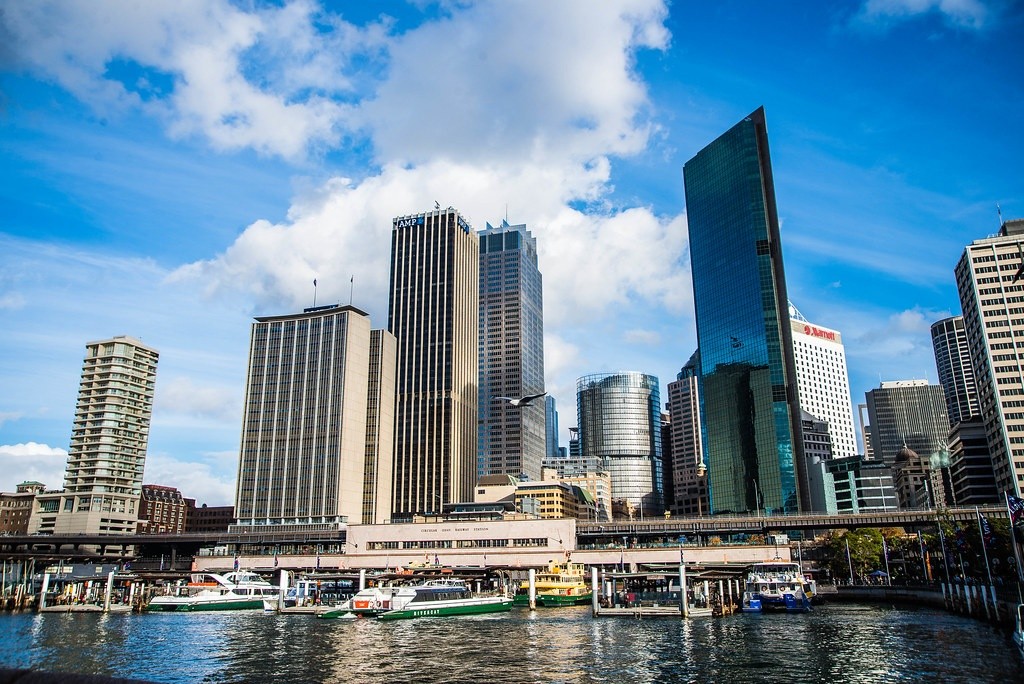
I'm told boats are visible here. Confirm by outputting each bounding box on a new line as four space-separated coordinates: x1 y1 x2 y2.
146 571 279 612
742 561 817 611
316 564 514 620
1014 604 1024 657
514 548 594 607
278 568 355 613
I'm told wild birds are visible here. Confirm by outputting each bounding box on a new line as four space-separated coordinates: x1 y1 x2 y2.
492 392 549 411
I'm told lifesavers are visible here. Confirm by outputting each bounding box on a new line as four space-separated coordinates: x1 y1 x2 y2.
766 578 772 582
783 575 787 581
750 576 757 582
780 586 785 591
788 576 792 581
370 601 374 607
376 601 381 607
762 586 767 591
